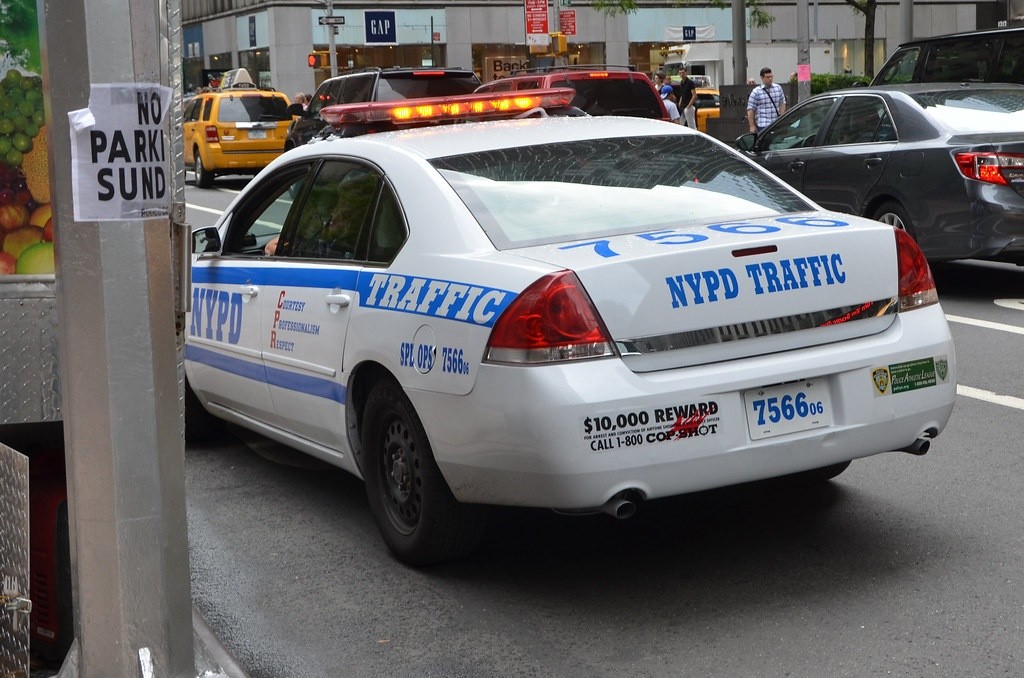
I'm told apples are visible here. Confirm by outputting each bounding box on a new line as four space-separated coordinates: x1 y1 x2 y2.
0 202 56 279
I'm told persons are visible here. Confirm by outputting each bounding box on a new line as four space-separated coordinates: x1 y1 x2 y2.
293 92 312 121
655 67 697 131
265 236 279 254
746 67 786 133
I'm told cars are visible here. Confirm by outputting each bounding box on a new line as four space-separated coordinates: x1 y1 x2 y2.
183 68 300 188
733 82 1024 263
184 113 961 570
694 87 720 134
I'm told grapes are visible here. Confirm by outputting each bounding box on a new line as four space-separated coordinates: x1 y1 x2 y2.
0 69 46 204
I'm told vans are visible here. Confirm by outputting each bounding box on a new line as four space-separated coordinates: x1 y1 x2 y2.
851 26 1024 88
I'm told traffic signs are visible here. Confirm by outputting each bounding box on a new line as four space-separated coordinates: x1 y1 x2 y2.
525 0 549 34
318 16 345 25
559 9 576 35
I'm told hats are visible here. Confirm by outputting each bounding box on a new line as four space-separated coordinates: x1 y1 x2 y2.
660 85 673 99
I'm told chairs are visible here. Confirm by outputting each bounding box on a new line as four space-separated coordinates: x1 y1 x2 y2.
297 175 404 261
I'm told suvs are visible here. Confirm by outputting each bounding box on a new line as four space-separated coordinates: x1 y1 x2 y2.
472 63 672 125
283 65 483 202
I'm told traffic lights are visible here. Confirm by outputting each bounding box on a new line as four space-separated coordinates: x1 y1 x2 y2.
307 54 327 69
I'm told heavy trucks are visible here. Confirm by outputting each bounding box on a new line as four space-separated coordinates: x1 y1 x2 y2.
660 42 836 89
